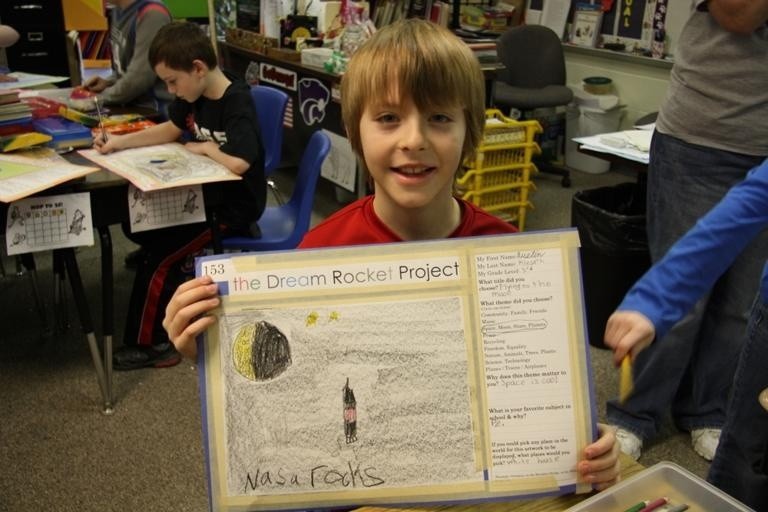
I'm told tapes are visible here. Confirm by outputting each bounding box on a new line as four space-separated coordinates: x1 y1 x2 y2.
583 77 612 94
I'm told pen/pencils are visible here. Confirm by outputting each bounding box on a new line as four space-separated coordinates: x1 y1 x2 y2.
94 96 108 143
623 497 690 512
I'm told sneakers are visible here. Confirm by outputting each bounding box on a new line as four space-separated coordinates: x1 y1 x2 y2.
113 343 180 370
617 428 721 460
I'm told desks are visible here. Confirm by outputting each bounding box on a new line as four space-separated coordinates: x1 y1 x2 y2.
571 122 656 179
216 40 374 202
0 145 222 415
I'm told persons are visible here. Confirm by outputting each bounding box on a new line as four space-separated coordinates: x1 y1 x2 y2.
79 0 179 267
597 0 768 465
92 19 267 370
162 16 622 494
0 25 22 83
599 153 768 512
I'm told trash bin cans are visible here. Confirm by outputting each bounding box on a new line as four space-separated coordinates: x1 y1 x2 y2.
571 180 652 349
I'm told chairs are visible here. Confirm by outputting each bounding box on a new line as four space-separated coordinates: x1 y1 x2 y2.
250 84 291 208
490 25 573 187
222 128 331 252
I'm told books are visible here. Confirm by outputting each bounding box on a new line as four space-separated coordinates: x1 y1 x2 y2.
0 89 53 153
363 1 452 35
